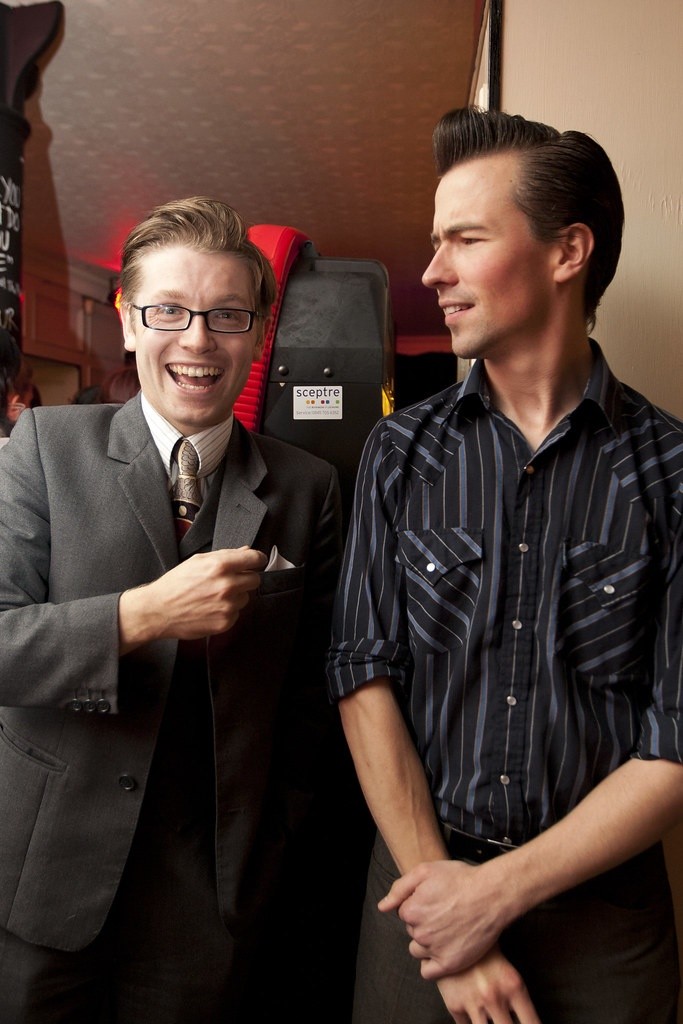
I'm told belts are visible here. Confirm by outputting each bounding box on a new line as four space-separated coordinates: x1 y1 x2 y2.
441 824 516 863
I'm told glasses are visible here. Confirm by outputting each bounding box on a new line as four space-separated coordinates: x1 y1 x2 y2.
133 305 259 334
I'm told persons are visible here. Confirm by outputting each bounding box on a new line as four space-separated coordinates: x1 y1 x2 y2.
0 326 141 448
0 196 346 1024
329 104 683 1024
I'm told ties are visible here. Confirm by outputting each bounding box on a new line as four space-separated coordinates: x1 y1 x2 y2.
167 441 203 543
